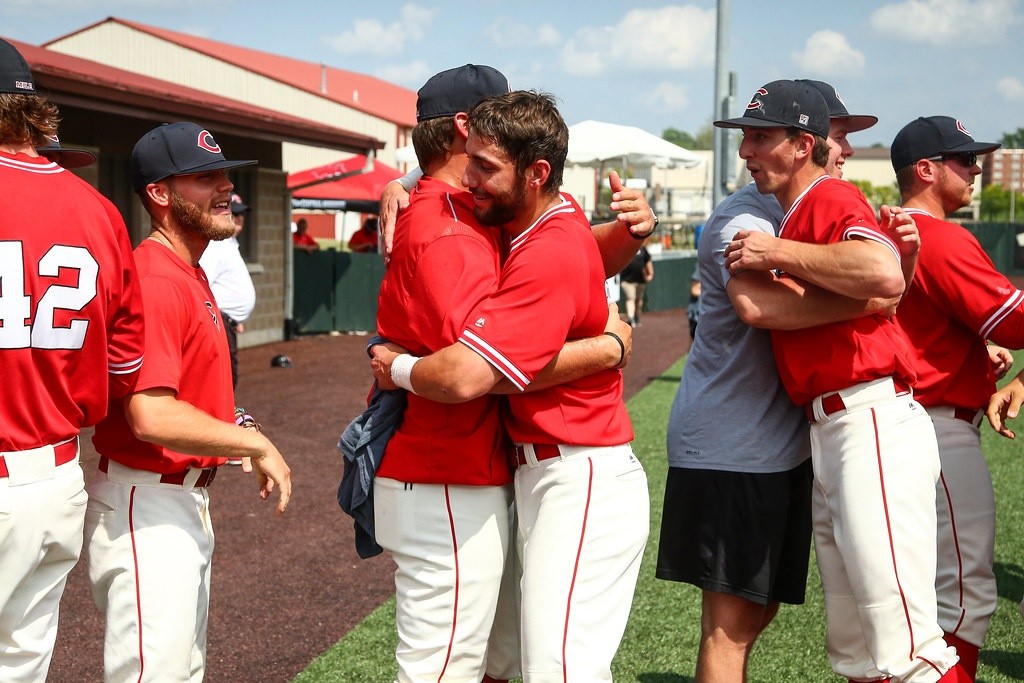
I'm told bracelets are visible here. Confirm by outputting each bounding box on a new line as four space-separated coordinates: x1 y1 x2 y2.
391 353 421 395
626 208 658 240
603 332 624 366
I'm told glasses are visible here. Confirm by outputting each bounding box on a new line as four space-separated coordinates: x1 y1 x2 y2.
913 150 976 167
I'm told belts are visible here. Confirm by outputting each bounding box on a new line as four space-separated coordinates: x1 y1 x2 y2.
804 377 911 423
221 313 237 328
954 407 985 429
509 443 560 470
0 435 77 477
98 455 217 488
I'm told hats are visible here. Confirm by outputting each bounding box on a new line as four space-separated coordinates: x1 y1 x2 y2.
130 122 259 194
0 37 37 95
891 115 1001 173
230 192 251 214
713 80 831 140
415 64 508 121
36 119 96 167
796 79 879 133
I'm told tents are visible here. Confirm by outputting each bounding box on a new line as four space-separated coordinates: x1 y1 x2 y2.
288 155 404 215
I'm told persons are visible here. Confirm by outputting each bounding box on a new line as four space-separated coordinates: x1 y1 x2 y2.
293 218 319 254
687 262 701 341
713 80 973 683
366 64 656 683
374 89 650 683
655 79 920 683
84 123 292 683
199 193 256 388
986 367 1024 439
620 245 655 328
888 115 1024 683
350 219 377 253
0 37 147 683
